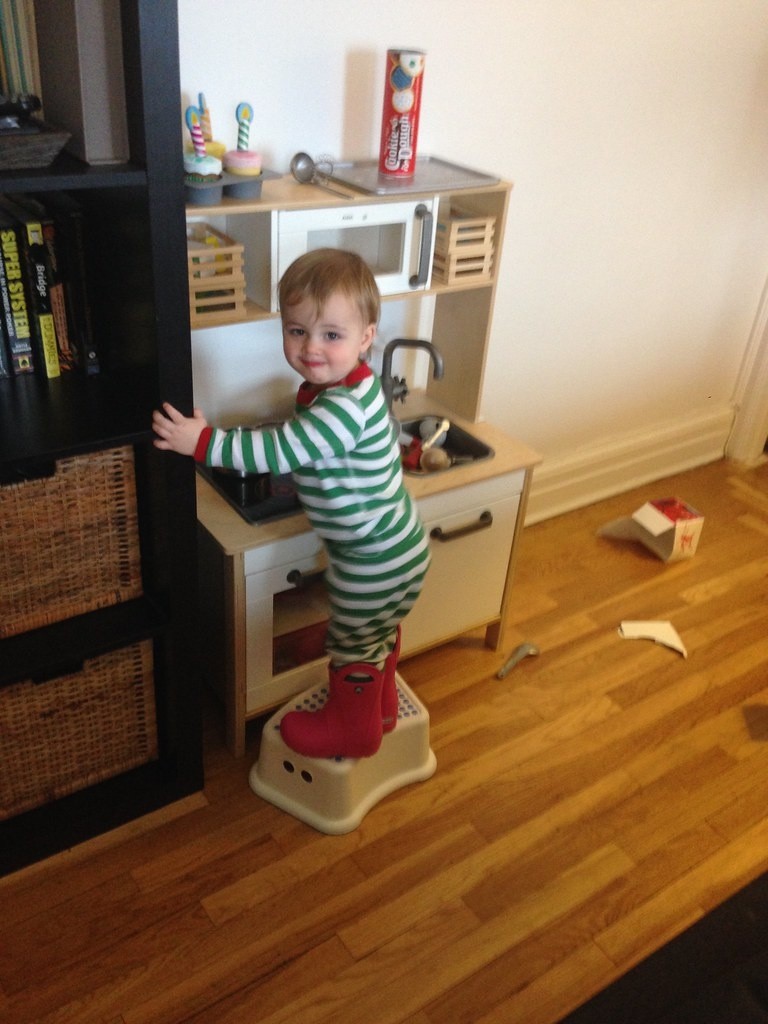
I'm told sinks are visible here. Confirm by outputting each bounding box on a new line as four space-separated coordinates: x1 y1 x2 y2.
392 413 496 479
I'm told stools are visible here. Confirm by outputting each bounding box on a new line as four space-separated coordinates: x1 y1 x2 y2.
246 664 435 836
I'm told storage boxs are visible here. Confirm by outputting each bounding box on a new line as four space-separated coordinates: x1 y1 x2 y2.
632 494 706 564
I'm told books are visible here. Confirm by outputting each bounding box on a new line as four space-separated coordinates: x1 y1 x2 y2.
0 193 102 378
0 0 45 118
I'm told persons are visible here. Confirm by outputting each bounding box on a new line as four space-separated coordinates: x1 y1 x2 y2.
153 248 431 756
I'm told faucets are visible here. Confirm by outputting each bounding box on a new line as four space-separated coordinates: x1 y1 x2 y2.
376 338 445 416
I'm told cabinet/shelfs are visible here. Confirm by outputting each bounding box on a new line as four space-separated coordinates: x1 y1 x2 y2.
186 155 543 759
0 0 208 892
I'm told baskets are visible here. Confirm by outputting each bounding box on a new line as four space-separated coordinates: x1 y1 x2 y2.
0 445 142 642
0 638 157 819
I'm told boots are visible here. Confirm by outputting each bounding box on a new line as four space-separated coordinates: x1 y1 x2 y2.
381 624 402 733
279 662 383 758
